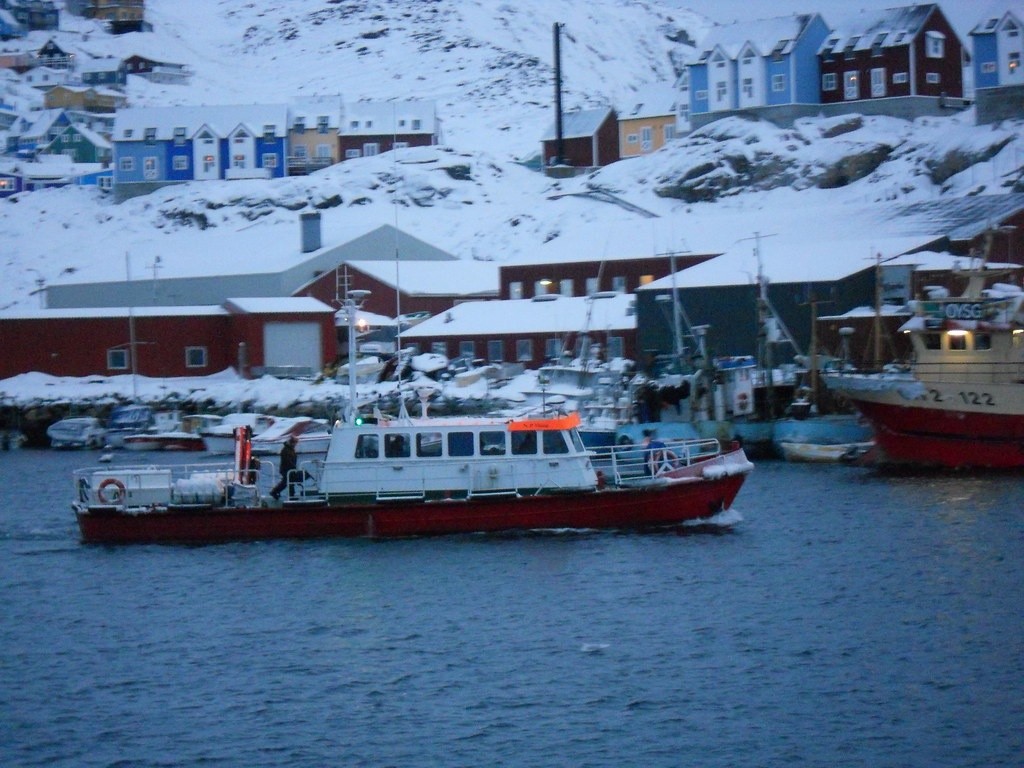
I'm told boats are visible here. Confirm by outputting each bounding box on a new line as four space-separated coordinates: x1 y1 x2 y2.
840 230 1024 467
72 104 753 542
780 438 877 464
47 413 333 455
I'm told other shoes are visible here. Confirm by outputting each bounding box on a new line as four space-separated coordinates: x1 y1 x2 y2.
270 490 279 501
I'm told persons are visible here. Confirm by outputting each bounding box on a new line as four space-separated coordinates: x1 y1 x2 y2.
388 435 405 457
641 437 669 475
269 435 298 500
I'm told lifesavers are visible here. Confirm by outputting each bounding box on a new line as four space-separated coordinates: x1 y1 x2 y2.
655 450 678 473
98 478 126 506
617 435 635 452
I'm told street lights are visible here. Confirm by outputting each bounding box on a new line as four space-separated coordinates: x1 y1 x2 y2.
554 22 577 164
346 290 371 424
